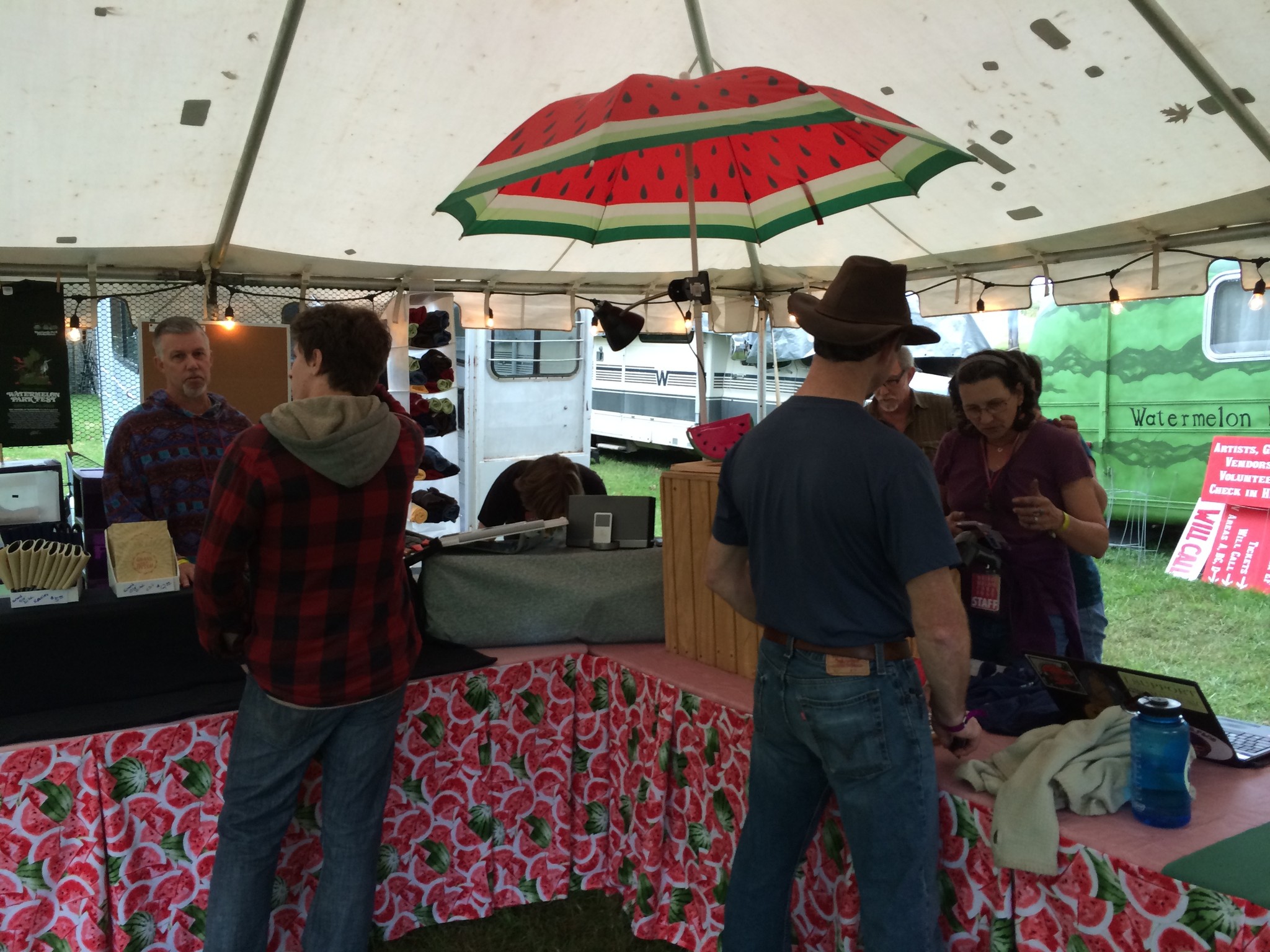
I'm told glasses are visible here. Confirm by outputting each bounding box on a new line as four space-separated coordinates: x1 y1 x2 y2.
883 369 905 388
960 391 1015 419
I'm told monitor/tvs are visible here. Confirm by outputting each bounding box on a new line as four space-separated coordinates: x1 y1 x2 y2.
0 459 66 532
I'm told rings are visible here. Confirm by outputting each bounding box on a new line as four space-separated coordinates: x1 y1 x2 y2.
1039 506 1044 515
1033 516 1038 524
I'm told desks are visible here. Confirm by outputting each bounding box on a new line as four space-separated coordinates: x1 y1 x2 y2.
0 581 1270 952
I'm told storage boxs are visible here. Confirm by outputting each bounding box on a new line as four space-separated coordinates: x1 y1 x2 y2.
656 456 762 679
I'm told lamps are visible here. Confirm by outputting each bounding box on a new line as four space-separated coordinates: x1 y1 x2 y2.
488 294 494 327
590 270 716 351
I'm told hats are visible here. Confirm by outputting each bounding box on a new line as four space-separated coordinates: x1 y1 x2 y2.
786 255 941 346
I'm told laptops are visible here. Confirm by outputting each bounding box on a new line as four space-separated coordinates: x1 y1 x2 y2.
1019 647 1270 766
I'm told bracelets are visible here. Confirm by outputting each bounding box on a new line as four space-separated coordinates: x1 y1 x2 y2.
177 559 190 565
931 709 988 732
1051 511 1070 536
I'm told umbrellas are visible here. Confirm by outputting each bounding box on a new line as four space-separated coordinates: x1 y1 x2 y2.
431 64 982 465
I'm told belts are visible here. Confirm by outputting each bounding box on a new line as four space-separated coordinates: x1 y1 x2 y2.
762 627 910 662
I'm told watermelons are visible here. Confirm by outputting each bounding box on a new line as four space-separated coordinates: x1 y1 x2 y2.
687 413 755 462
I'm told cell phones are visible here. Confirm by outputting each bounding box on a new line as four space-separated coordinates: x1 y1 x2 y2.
955 520 991 530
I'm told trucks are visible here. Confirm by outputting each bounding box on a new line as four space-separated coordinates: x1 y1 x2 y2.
596 247 1270 545
0 277 140 506
94 292 597 547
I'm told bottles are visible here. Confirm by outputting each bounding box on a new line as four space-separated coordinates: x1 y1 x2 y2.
1131 697 1191 829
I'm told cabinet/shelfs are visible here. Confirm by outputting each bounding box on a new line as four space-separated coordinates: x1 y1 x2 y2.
71 468 104 589
381 292 463 534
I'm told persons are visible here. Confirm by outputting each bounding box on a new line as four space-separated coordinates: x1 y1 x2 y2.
931 351 1109 666
103 317 253 587
192 299 424 952
477 454 606 541
864 346 960 461
698 255 980 952
